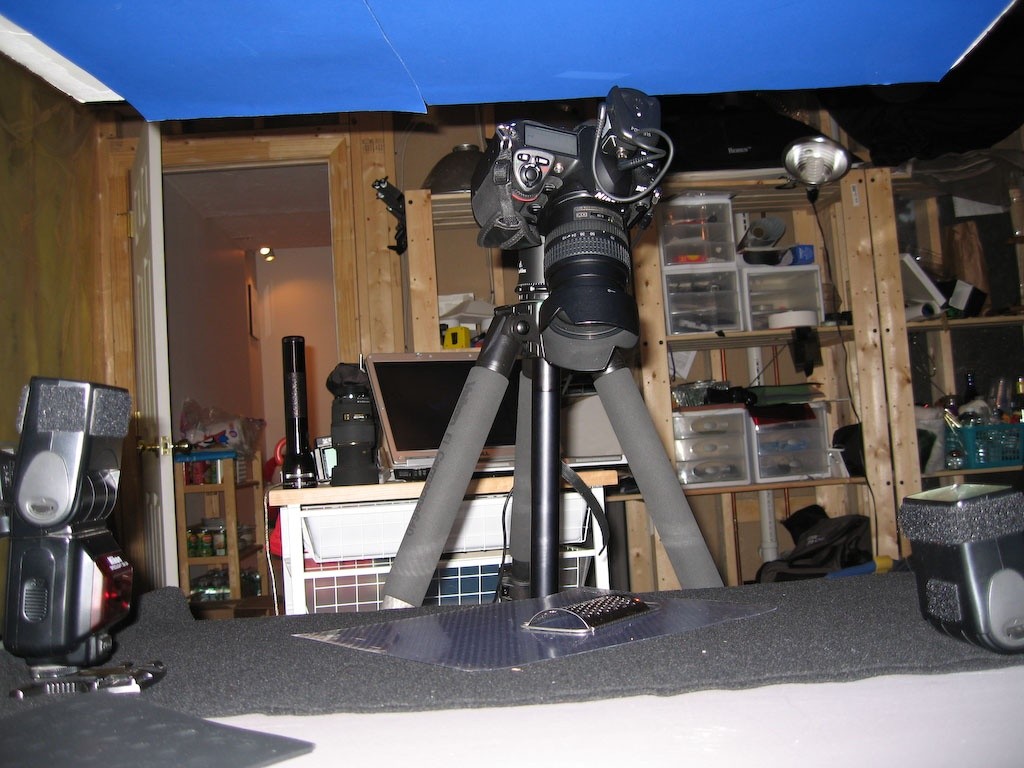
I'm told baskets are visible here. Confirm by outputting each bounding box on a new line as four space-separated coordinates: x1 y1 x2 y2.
943 424 1024 471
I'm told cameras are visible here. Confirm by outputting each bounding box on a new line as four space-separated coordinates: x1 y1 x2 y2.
470 83 674 372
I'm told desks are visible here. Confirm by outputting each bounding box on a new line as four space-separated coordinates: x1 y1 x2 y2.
263 468 620 616
0 569 1024 768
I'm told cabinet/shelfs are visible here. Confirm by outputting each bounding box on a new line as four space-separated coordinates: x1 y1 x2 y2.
862 124 1024 562
625 165 902 597
173 444 272 604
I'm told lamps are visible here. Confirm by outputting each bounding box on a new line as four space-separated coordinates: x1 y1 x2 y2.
775 126 855 194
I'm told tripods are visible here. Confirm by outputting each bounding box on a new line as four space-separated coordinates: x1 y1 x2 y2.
373 238 726 610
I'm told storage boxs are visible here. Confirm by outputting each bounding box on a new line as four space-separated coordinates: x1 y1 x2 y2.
189 599 277 621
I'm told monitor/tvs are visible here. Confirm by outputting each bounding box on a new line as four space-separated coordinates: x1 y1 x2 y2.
364 350 629 475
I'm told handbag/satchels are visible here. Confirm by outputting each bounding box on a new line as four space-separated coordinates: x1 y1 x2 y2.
787 513 873 570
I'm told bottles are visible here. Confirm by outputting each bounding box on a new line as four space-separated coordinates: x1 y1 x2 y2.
1010 376 1024 422
963 371 978 404
988 380 1009 422
